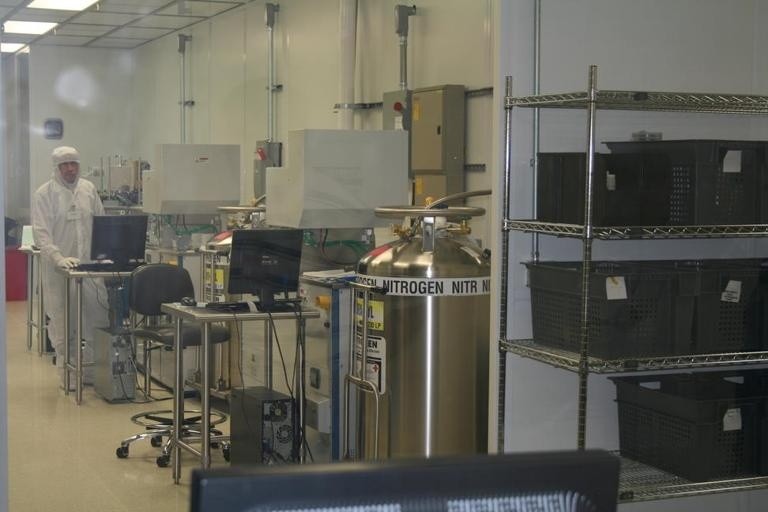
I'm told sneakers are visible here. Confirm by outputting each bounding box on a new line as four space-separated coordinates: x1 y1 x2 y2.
82 374 96 384
61 375 83 390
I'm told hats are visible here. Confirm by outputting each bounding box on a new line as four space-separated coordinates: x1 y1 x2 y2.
52 145 81 188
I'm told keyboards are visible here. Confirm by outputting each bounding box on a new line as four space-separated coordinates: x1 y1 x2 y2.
77 262 147 272
206 301 301 313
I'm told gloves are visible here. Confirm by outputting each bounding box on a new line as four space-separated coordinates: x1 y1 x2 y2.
49 252 80 269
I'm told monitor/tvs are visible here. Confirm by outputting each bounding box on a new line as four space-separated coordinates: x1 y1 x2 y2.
228 229 303 313
90 215 148 271
190 449 621 511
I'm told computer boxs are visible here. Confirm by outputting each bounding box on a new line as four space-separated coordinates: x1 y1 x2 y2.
229 386 300 466
93 326 136 403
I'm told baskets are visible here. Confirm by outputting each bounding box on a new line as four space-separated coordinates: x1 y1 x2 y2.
631 258 768 353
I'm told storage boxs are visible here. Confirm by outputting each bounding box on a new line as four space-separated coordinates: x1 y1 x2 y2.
622 258 758 357
535 152 645 229
715 257 767 348
700 371 768 476
737 140 768 230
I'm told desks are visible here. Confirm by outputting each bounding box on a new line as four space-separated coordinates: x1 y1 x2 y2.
160 303 319 486
20 245 140 405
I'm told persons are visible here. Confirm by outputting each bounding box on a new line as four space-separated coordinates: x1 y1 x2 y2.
28 146 104 391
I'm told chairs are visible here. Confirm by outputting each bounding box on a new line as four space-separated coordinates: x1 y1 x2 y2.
114 263 228 466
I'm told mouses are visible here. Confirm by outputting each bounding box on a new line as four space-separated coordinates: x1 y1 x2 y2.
181 296 197 306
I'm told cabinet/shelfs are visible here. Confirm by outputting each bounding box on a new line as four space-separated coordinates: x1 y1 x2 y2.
497 77 768 502
